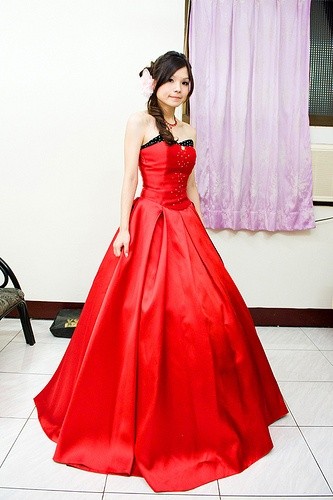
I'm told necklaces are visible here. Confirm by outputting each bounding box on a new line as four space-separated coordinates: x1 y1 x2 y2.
163 115 177 130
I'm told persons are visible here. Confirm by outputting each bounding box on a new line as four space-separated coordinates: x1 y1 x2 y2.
107 51 223 447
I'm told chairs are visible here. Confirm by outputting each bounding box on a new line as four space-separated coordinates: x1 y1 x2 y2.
0 257 36 346
